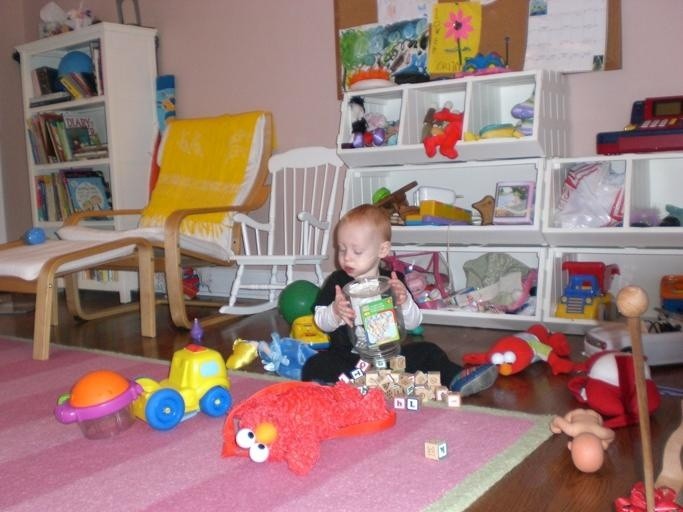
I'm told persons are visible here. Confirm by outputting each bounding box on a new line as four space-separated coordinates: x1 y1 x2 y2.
300 205 498 396
551 408 616 473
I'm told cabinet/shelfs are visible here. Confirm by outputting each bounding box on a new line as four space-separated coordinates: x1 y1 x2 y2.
339 157 549 244
14 23 160 304
384 246 545 332
543 247 683 338
542 151 683 249
337 70 568 169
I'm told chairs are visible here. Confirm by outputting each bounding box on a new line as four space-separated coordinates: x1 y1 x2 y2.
66 111 279 324
219 146 344 318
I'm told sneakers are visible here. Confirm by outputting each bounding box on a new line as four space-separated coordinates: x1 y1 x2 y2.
449 364 498 397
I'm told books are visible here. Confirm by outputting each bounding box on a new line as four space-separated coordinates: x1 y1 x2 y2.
25 42 112 223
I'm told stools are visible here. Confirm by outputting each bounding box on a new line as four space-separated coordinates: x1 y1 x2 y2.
0 236 158 362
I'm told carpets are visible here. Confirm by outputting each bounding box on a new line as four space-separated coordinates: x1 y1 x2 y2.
0 335 562 512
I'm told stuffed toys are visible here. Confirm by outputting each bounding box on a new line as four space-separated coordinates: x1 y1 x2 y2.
222 381 396 473
569 350 660 429
341 98 368 148
463 325 575 377
424 100 463 158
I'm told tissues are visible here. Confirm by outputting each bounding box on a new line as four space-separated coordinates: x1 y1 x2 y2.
38 1 93 40
